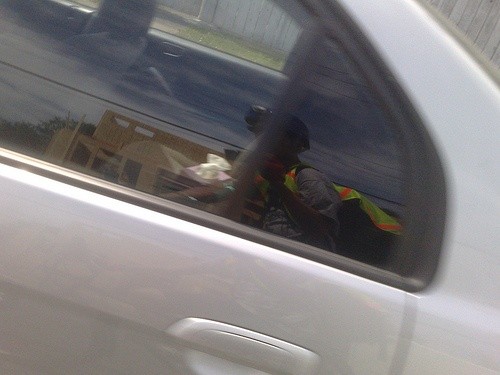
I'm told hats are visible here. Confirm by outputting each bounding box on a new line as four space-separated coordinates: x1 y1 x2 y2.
245 104 311 149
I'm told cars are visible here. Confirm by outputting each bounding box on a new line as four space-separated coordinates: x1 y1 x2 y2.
2 0 500 373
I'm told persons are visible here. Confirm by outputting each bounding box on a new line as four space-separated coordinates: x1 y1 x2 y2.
223 109 341 257
